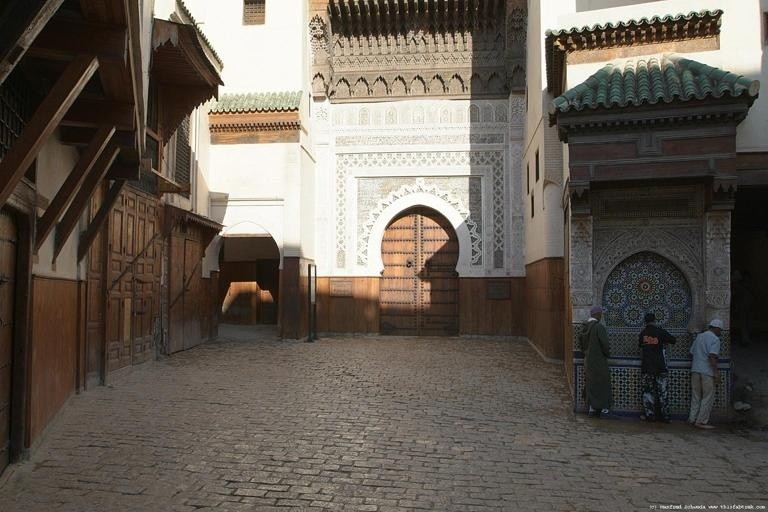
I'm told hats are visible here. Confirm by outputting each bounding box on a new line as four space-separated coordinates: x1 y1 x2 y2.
710 320 724 330
591 307 601 315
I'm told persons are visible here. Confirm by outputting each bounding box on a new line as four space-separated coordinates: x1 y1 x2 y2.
685 319 723 429
577 305 618 419
638 312 677 424
